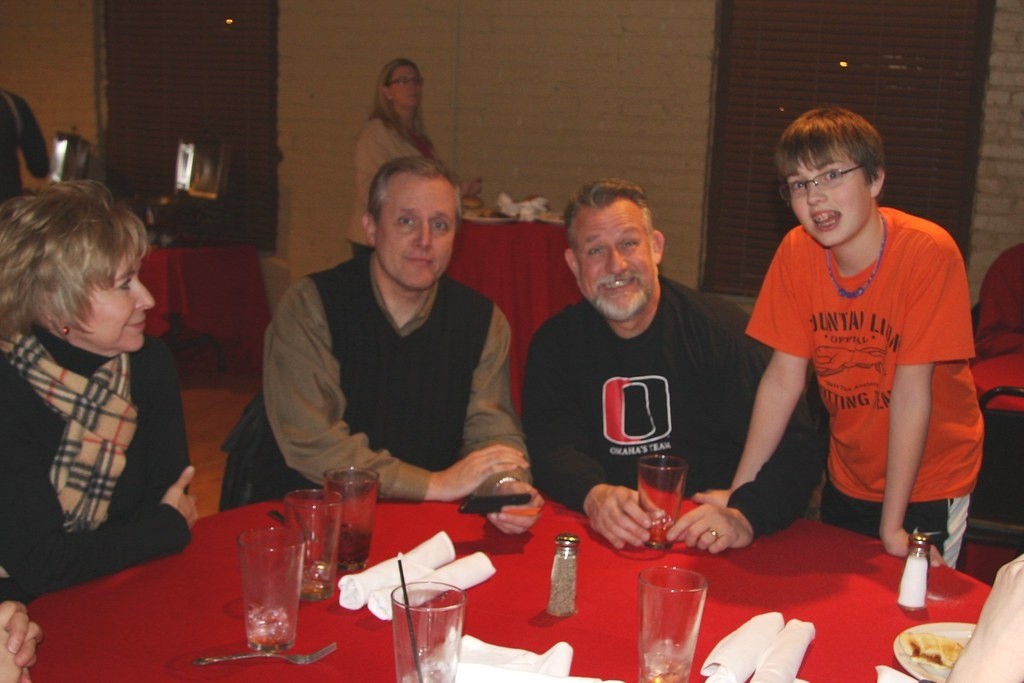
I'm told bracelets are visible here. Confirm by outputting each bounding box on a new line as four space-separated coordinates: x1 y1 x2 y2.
495 475 515 492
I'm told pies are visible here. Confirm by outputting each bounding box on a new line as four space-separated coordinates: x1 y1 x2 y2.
899 631 964 670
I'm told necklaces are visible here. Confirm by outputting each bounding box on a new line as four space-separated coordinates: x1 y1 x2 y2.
826 209 886 298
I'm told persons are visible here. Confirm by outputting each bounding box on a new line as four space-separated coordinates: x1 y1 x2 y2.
692 106 983 566
1 600 41 683
346 57 484 259
259 155 543 532
978 240 1024 360
942 552 1021 682
0 90 49 201
521 179 821 552
1 180 199 595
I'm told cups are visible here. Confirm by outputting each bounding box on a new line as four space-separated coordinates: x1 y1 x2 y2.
638 566 708 682
283 488 343 602
391 582 465 683
237 526 306 652
635 454 686 549
327 469 379 570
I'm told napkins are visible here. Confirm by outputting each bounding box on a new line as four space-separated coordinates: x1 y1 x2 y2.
369 553 497 622
338 530 455 611
750 620 816 683
444 635 625 683
700 610 787 683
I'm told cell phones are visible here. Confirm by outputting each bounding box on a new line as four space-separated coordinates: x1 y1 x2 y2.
459 494 530 514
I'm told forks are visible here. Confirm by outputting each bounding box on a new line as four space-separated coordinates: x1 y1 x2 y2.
193 642 337 666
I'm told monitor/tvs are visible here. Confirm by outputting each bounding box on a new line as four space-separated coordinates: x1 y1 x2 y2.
49 129 89 183
174 137 201 192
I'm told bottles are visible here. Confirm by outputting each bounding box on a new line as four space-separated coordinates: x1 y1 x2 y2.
519 197 536 222
547 532 579 618
897 533 930 612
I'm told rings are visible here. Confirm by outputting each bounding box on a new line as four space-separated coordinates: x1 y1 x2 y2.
711 529 718 537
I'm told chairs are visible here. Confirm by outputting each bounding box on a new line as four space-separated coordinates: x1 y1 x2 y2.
961 386 1024 557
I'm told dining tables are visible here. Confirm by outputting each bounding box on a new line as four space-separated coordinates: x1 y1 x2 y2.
140 234 272 386
447 221 582 421
970 351 1023 411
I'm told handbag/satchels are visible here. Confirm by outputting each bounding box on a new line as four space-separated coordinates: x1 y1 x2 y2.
15 148 53 196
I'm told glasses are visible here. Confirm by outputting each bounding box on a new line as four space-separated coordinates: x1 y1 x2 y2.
780 164 863 201
385 75 425 88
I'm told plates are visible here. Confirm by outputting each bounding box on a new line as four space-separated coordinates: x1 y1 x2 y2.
894 623 979 682
463 213 516 223
537 213 567 224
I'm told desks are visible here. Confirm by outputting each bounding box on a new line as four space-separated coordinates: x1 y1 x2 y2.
20 489 993 683
